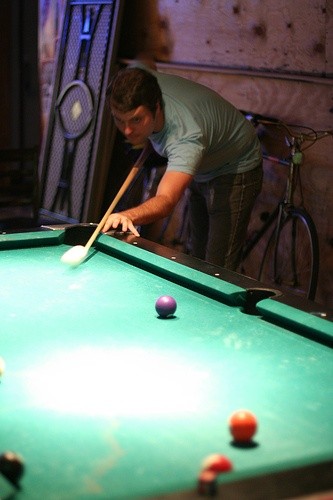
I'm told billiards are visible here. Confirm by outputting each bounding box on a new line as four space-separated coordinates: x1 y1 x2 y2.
0 451 26 483
59 245 89 268
230 410 258 442
201 452 233 475
155 295 177 319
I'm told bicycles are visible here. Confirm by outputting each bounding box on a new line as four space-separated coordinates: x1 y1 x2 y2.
183 110 333 302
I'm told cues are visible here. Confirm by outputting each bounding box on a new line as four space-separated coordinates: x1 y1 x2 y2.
83 144 151 250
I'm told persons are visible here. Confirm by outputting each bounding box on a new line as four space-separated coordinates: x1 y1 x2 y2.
92 62 264 273
